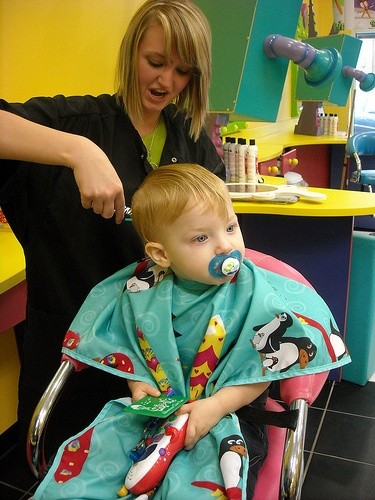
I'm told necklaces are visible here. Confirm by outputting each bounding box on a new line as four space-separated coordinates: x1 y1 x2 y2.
131 119 158 169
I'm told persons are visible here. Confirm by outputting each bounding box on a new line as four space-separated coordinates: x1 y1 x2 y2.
30 162 352 500
0 0 225 500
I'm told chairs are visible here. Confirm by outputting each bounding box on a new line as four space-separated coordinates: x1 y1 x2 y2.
27 248 330 500
346 130 375 193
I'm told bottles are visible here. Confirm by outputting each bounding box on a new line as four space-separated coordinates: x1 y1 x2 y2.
319 113 338 135
219 135 259 181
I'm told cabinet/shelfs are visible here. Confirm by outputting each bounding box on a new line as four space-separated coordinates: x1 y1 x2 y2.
0 128 375 436
